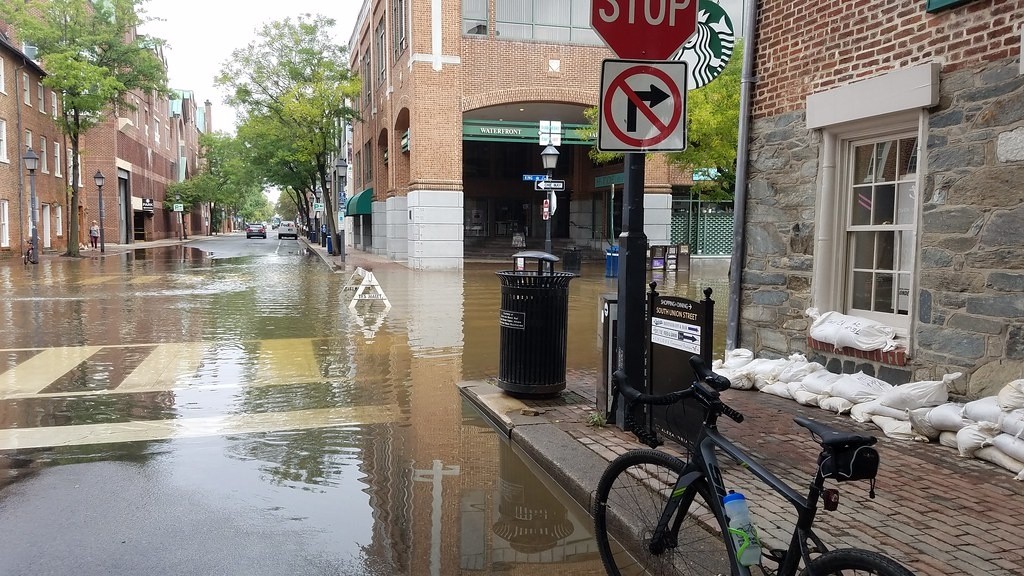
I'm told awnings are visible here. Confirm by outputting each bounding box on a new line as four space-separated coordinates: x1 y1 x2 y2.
344 188 372 216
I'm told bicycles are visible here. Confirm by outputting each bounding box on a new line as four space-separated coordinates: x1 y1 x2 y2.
592 353 917 576
23 238 36 265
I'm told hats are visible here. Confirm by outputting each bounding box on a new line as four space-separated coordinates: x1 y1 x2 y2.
92 220 96 222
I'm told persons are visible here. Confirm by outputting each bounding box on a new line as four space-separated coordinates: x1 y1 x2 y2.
28 235 41 261
88 220 100 251
296 222 309 239
184 222 187 232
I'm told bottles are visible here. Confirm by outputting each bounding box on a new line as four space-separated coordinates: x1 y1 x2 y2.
723 490 760 566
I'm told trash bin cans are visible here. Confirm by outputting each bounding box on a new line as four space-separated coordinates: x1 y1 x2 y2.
605 249 619 278
561 246 582 277
327 235 341 255
310 232 318 243
495 251 582 401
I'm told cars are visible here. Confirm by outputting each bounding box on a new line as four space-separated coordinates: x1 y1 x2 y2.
278 220 298 240
246 223 266 238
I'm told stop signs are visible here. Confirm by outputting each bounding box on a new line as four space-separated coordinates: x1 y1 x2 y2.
589 0 699 60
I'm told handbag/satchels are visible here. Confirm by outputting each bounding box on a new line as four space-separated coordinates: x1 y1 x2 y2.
90 227 91 234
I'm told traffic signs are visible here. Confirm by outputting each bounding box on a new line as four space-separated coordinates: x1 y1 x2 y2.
535 180 565 192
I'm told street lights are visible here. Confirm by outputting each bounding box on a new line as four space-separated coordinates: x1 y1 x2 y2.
93 168 105 253
221 205 225 234
175 191 183 241
336 155 348 263
205 201 209 236
22 146 41 264
540 139 560 272
